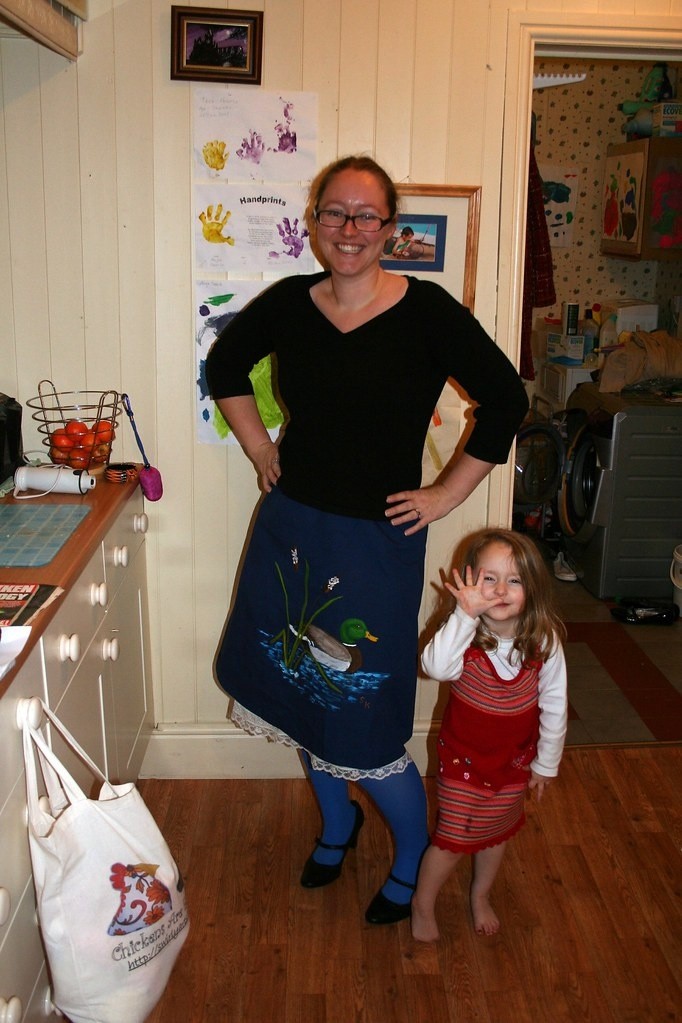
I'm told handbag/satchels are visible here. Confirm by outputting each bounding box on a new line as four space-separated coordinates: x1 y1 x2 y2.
21 696 190 1023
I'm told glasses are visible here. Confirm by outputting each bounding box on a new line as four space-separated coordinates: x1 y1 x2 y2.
312 205 393 233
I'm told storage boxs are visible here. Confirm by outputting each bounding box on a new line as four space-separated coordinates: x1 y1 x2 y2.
546 331 585 365
651 102 682 138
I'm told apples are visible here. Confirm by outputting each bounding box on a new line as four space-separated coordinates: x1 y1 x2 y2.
50 421 113 468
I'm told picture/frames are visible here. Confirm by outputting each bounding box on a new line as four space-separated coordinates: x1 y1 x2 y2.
380 183 483 317
171 4 264 86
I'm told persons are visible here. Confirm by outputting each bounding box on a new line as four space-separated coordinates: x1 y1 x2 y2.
411 528 568 945
391 226 413 256
201 155 530 926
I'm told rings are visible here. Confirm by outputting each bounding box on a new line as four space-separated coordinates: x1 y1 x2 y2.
271 459 279 463
414 509 420 519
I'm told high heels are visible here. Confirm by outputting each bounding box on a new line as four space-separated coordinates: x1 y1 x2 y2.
300 800 364 889
366 874 418 924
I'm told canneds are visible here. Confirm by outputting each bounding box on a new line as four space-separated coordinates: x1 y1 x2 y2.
561 302 579 335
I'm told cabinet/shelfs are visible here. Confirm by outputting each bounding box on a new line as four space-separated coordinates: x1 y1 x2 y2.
0 463 156 1023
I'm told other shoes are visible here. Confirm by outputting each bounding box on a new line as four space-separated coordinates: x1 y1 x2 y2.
552 552 577 581
610 601 679 625
